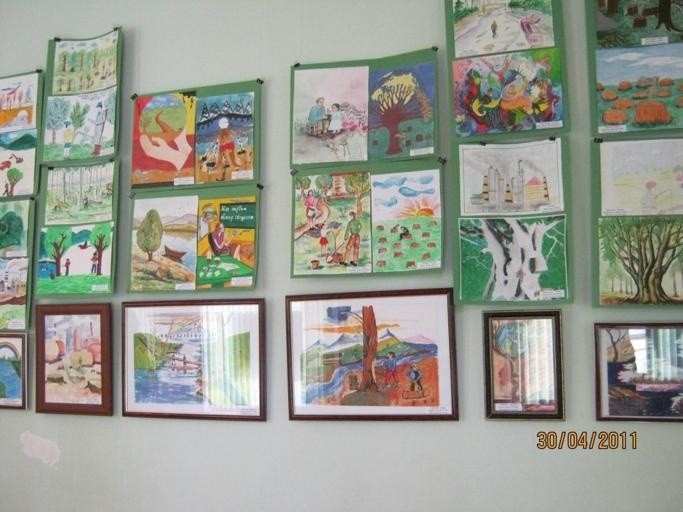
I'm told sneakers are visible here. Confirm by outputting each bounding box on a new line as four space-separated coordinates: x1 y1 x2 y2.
339 260 359 267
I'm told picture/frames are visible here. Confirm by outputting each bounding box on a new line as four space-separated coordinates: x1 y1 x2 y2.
35 302 117 417
482 308 566 423
275 286 460 423
1 332 29 411
118 297 267 423
592 320 683 422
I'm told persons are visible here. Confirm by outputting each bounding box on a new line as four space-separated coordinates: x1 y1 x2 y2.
318 222 330 257
3 271 9 292
308 97 329 135
90 250 98 276
211 222 241 262
328 103 343 134
62 120 73 159
62 257 70 276
406 363 424 391
399 225 409 240
491 20 498 36
83 194 88 207
341 211 362 268
382 351 400 387
4 183 9 196
673 165 683 191
632 181 669 213
90 100 105 155
213 117 246 181
140 127 192 172
304 189 316 231
